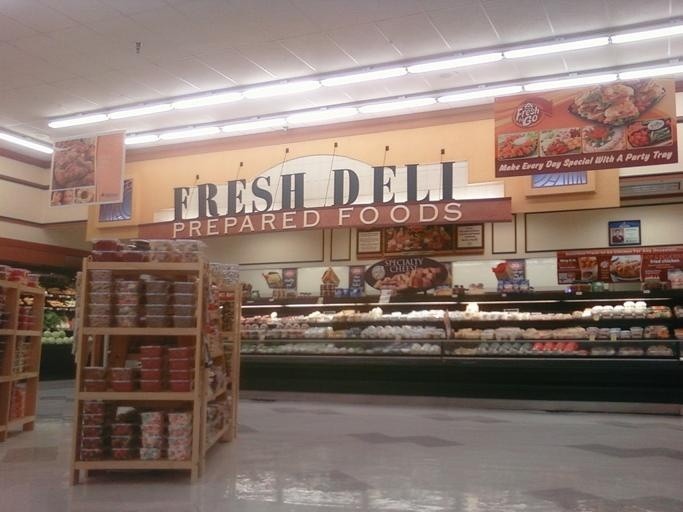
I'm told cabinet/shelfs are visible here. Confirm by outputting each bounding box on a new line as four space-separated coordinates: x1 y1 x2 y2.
1 265 47 440
241 292 683 356
70 258 240 485
0 18 683 155
40 290 77 381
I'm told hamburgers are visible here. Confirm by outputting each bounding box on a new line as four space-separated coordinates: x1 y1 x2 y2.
574 79 662 125
